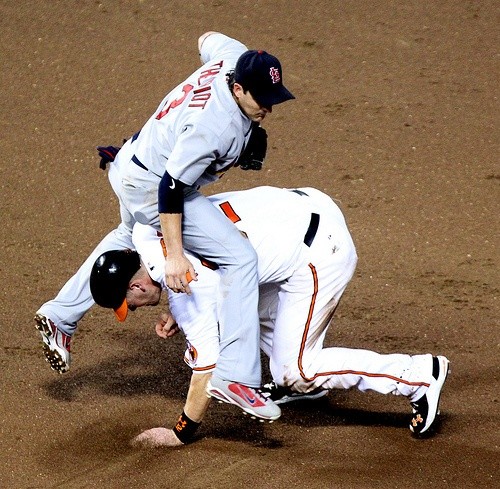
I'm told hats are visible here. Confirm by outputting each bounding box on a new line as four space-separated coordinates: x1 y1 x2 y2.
233 49 296 108
90 250 140 322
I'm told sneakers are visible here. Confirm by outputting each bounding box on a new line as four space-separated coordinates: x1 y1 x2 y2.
206 375 281 423
262 379 328 404
34 315 71 375
409 353 449 435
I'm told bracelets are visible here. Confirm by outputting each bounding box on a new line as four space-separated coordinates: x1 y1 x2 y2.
172 410 202 445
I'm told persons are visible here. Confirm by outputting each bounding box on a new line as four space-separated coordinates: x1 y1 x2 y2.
90 185 451 449
34 30 296 424
